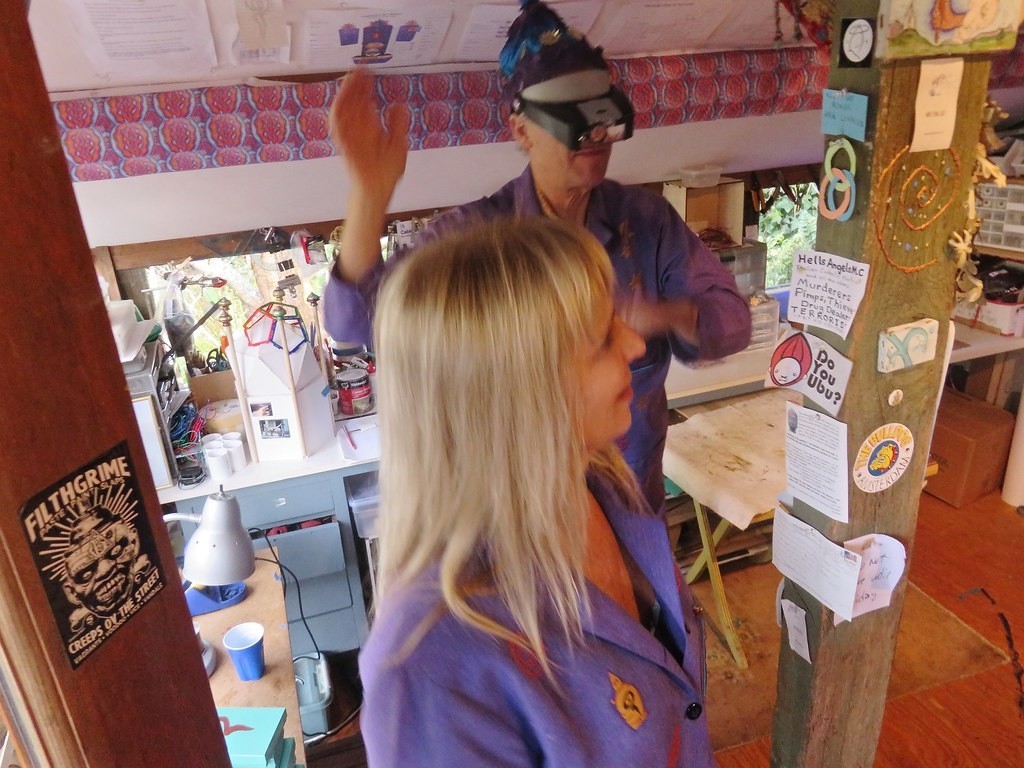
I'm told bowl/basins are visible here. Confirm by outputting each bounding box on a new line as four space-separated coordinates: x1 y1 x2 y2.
680 165 723 187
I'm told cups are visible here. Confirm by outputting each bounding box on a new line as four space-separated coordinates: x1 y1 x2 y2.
223 622 266 680
222 432 242 441
203 442 222 467
208 450 231 480
223 440 246 470
201 432 221 445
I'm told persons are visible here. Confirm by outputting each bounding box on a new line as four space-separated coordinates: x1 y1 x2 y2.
357 216 721 768
319 33 755 546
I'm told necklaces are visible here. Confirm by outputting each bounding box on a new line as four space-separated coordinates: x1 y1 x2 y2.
535 189 559 220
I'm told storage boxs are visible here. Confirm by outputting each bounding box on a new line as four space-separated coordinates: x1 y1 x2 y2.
954 299 1024 335
345 471 379 538
924 387 1016 510
972 183 1024 253
245 374 333 457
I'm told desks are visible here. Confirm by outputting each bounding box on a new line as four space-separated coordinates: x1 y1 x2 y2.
131 317 1024 505
153 546 303 768
645 388 939 669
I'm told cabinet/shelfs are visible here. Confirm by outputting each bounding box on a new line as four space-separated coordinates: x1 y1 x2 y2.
176 459 370 656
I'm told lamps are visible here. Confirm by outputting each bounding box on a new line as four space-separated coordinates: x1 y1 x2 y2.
160 484 256 677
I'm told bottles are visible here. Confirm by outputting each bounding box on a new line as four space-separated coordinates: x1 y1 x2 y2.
368 362 377 396
